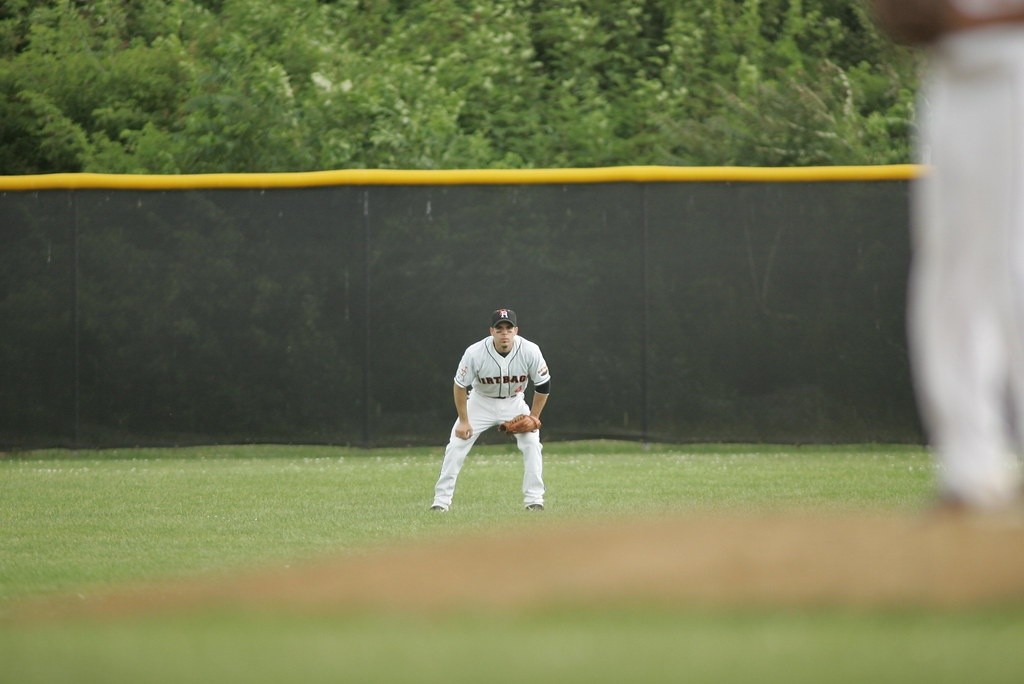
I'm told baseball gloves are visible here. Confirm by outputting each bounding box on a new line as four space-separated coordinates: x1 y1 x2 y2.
498 414 542 435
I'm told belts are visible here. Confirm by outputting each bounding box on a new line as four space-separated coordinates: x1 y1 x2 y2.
493 396 516 399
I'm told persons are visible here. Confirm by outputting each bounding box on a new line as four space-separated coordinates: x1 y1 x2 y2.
430 309 551 512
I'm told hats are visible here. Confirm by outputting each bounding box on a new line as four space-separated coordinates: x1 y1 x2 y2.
491 309 517 327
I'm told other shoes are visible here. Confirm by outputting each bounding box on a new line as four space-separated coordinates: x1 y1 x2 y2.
430 506 445 512
526 503 543 510
